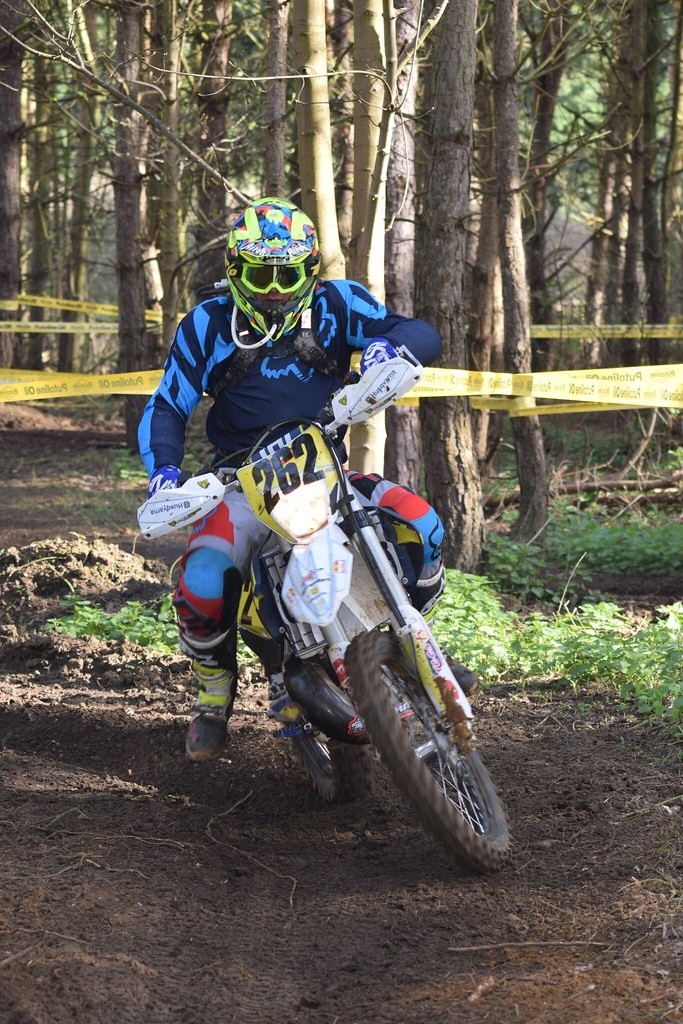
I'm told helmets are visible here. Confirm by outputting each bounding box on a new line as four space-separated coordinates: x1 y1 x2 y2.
225 197 320 342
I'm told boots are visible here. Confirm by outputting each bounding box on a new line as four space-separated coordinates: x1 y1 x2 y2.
415 561 480 697
179 623 238 762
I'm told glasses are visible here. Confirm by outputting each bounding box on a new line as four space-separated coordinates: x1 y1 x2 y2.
230 259 318 294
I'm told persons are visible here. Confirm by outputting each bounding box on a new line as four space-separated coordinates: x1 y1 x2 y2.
137 197 480 761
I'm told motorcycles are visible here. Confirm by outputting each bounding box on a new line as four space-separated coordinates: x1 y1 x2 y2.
136 345 514 873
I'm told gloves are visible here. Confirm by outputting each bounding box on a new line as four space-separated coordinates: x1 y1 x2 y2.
147 464 183 499
360 338 397 375
